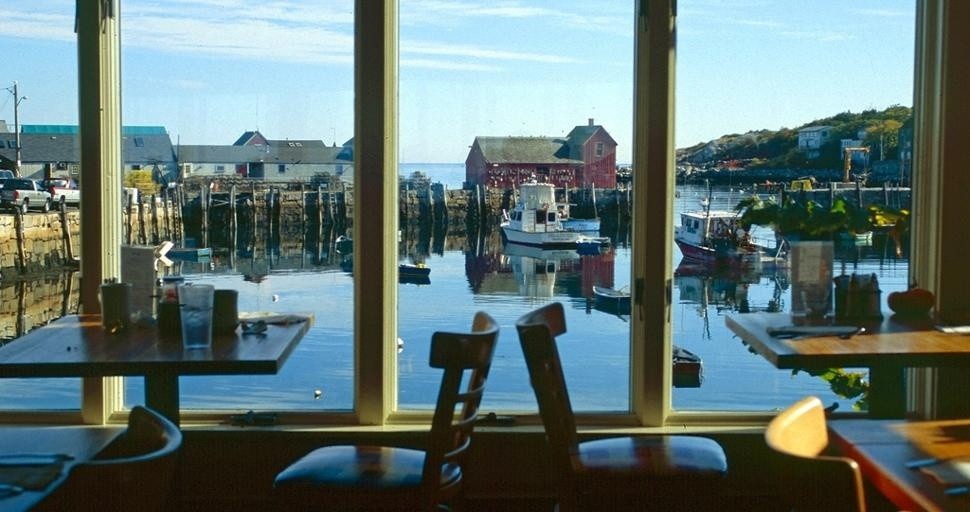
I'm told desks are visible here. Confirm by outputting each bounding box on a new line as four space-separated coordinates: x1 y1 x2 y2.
1 419 129 509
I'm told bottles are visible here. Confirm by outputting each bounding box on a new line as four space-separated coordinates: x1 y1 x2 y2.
157 275 185 326
833 271 884 324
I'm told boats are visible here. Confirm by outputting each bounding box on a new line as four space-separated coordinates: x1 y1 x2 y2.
673 343 702 373
592 284 631 308
674 184 790 272
499 245 585 297
673 263 791 340
341 252 430 285
499 180 612 245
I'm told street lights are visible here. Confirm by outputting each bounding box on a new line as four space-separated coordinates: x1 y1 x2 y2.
14 84 27 177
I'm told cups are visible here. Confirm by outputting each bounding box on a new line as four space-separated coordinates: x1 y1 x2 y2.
99 283 133 332
176 282 237 349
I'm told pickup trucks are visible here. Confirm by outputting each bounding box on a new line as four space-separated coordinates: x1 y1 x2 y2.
0 169 81 215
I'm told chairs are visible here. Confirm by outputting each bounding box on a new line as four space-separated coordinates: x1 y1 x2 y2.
74 404 185 508
513 303 732 508
273 312 502 507
760 396 865 509
